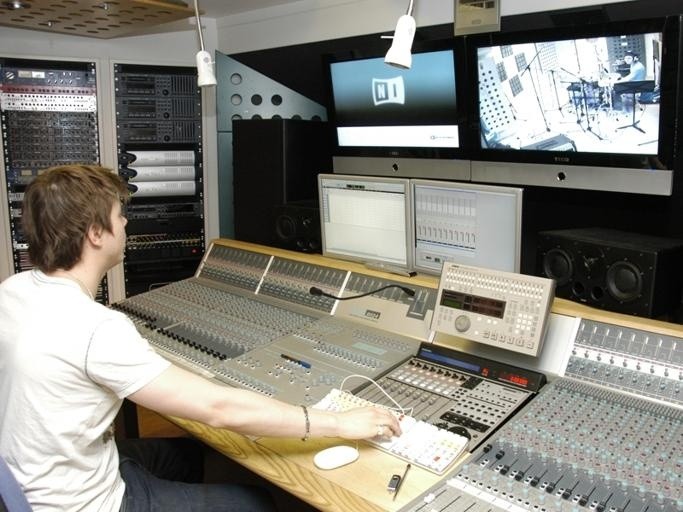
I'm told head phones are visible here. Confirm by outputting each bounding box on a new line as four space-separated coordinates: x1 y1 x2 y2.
626 51 639 62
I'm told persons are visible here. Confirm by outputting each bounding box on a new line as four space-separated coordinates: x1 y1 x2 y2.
0 164 403 512
614 51 646 84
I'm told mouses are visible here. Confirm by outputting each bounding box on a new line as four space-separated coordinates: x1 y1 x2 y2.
313 446 359 470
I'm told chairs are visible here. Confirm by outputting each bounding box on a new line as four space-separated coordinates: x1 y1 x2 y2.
2 462 34 512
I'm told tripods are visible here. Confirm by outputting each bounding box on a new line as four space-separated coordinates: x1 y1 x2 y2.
617 93 646 134
520 48 602 140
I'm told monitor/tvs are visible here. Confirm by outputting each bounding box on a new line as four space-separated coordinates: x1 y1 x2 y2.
471 15 683 196
322 34 471 181
410 180 524 276
318 174 411 271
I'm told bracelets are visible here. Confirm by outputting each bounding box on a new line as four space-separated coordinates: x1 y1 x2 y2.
300 404 311 442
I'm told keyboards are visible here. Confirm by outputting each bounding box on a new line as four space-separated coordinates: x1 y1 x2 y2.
312 389 470 476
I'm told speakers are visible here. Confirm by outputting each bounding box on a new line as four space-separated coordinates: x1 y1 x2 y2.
283 118 335 199
232 119 286 246
535 229 683 319
263 200 320 254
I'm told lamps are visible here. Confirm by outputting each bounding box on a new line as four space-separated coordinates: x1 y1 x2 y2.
195 0 219 88
386 1 416 68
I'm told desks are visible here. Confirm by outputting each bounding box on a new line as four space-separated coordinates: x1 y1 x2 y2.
133 238 683 512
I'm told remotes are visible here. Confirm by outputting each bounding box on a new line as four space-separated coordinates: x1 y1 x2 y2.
364 262 417 277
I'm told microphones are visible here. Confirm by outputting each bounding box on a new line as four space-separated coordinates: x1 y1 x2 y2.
310 285 415 300
